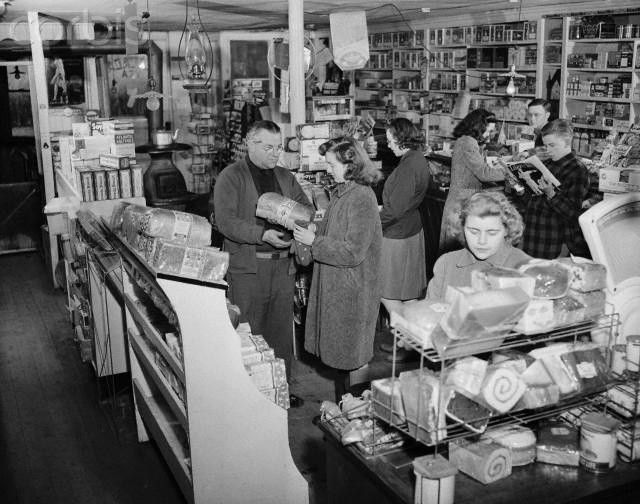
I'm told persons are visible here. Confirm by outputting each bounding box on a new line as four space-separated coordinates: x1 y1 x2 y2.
427 191 529 297
214 120 317 411
379 118 429 324
513 98 555 162
440 109 510 251
294 136 382 400
512 118 591 261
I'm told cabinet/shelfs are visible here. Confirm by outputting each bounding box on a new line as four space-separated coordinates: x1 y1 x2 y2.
101 217 309 504
426 18 544 125
538 17 562 133
55 168 146 378
353 29 427 129
562 17 640 166
368 303 640 470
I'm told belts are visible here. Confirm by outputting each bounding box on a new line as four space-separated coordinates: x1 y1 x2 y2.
256 252 290 260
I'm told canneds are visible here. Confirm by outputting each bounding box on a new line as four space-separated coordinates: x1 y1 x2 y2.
579 428 617 473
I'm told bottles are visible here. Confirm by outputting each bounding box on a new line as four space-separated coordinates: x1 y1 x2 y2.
627 335 640 373
611 344 627 377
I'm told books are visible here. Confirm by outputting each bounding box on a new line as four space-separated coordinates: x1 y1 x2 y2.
498 156 561 197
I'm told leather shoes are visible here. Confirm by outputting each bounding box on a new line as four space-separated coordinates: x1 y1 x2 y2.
379 343 400 353
289 394 305 408
313 415 321 424
386 350 408 363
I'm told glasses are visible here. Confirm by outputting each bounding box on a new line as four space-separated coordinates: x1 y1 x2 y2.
258 144 284 152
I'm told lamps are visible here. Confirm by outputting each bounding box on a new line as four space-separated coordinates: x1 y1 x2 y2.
177 0 214 93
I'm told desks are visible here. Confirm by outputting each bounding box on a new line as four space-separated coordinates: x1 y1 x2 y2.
312 412 640 504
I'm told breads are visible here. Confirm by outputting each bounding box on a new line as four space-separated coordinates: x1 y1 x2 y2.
537 421 579 469
139 207 212 247
256 191 314 230
372 258 614 438
121 204 145 235
448 437 512 482
110 201 131 230
127 232 154 260
152 240 229 282
480 424 536 466
